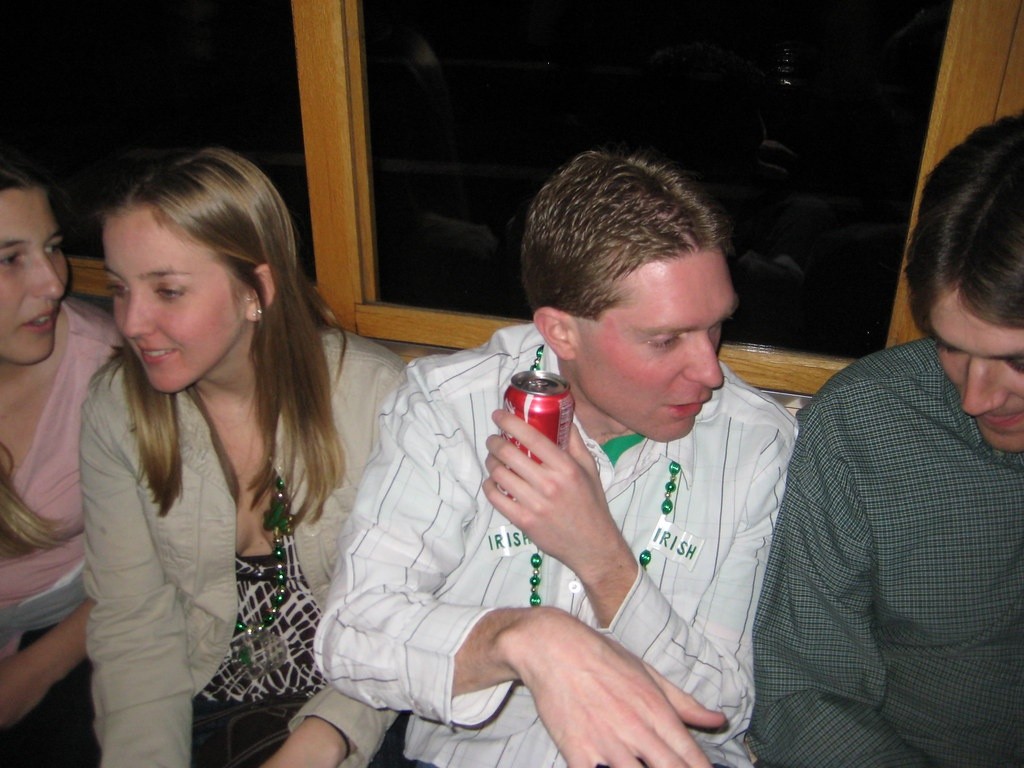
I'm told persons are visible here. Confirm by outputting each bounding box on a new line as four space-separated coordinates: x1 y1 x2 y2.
309 148 803 768
78 144 415 768
365 0 955 358
749 112 1024 768
0 156 124 768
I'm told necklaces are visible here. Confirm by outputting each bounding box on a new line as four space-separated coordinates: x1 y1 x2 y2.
231 477 296 680
527 337 683 607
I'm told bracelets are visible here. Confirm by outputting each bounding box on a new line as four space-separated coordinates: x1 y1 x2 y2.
304 716 350 760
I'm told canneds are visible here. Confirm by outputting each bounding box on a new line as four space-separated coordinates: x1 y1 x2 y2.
493 369 575 502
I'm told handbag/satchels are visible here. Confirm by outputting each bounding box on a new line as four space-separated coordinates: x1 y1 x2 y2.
193 693 312 768
